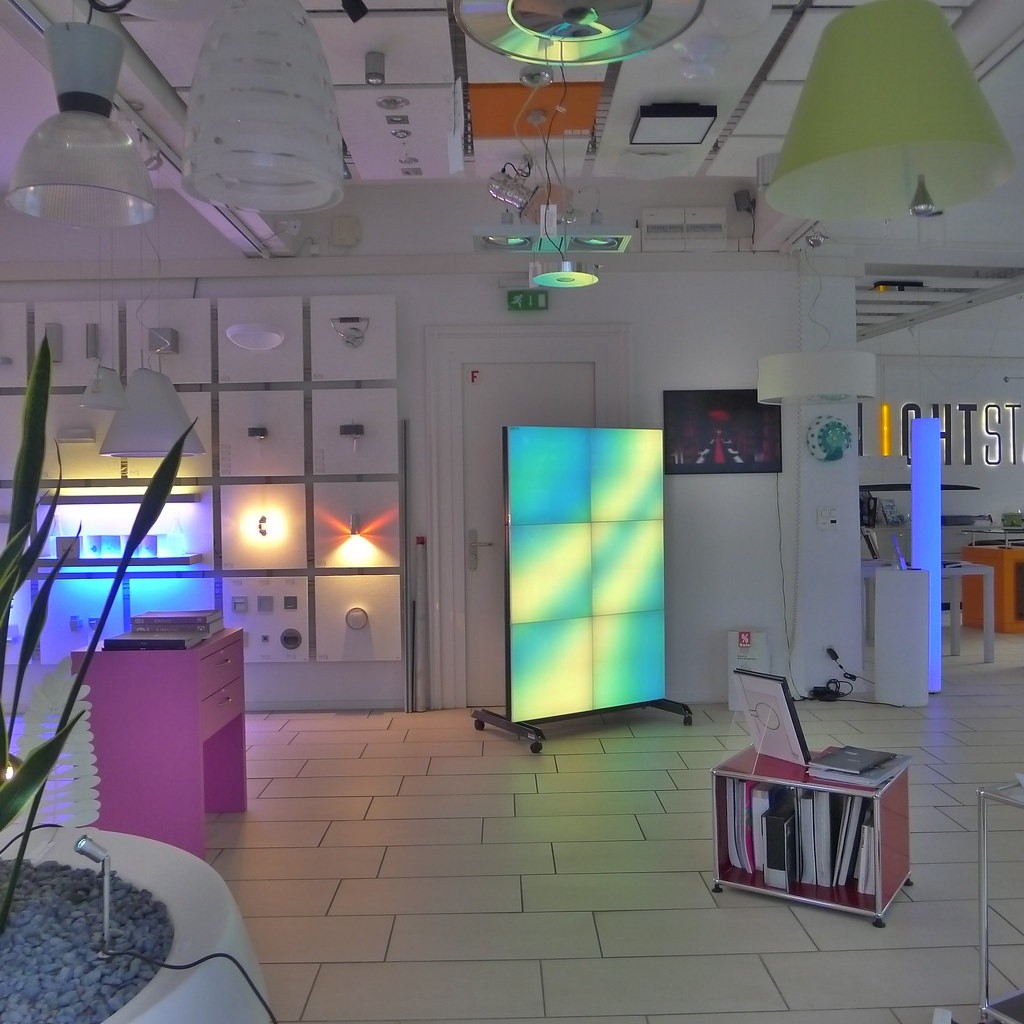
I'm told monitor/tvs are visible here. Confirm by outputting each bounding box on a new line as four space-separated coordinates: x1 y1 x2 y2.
663 390 782 474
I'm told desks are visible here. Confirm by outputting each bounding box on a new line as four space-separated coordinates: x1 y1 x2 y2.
70 627 250 861
941 561 996 664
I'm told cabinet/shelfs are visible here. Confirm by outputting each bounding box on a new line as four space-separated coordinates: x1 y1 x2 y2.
37 493 204 566
710 742 912 929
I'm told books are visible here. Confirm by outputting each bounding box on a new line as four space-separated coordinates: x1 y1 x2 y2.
734 668 811 763
808 745 913 789
101 610 225 650
726 777 877 896
942 561 962 568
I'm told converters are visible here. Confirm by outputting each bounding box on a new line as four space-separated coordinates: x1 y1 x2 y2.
814 686 830 697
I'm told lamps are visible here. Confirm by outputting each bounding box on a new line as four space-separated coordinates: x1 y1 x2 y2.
755 237 879 405
78 230 128 412
8 24 158 231
258 516 271 540
765 0 1018 236
364 52 387 85
99 142 205 460
342 0 368 23
183 0 344 213
450 1 705 292
349 513 361 535
